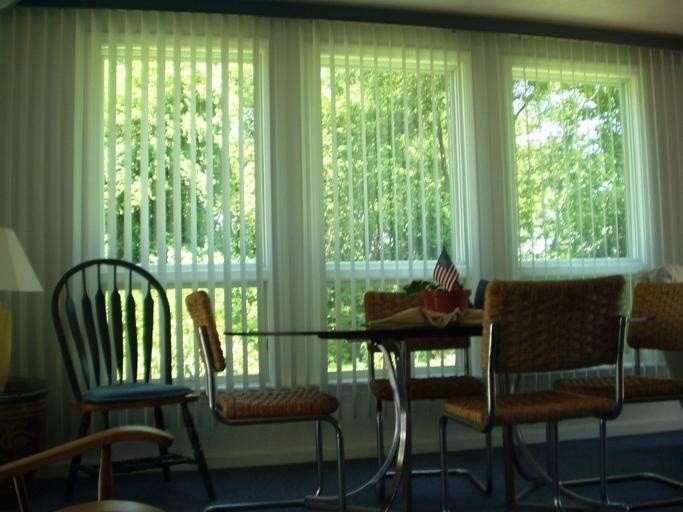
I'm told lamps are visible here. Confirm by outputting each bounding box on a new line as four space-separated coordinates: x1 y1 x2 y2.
0 223 45 396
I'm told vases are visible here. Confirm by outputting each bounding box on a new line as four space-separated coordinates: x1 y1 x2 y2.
419 290 471 314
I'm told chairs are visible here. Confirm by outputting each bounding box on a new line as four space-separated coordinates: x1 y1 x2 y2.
1 424 174 511
545 278 683 512
185 289 349 512
435 273 626 512
50 257 219 509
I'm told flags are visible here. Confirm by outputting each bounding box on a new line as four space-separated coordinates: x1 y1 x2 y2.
433 247 459 293
